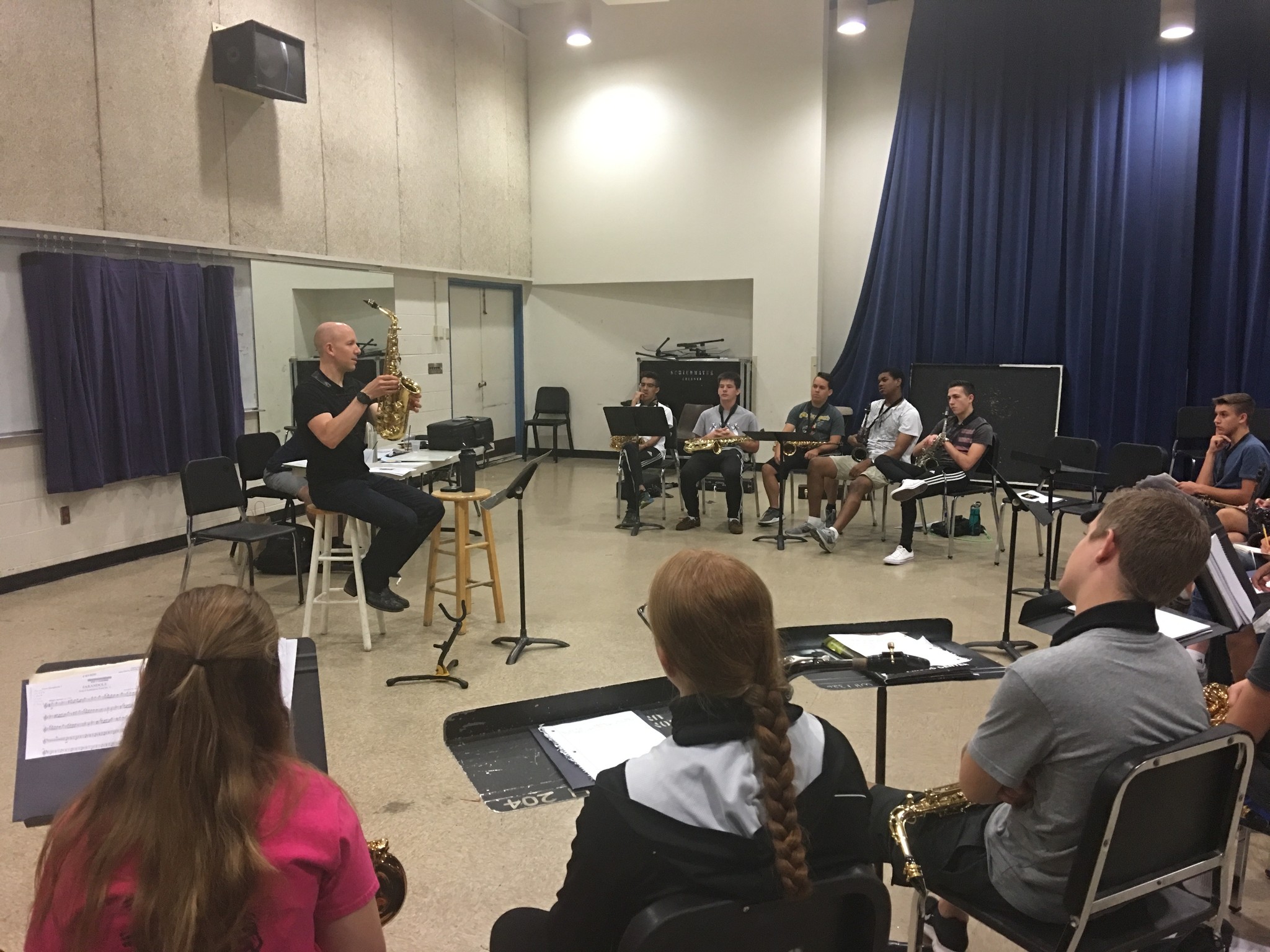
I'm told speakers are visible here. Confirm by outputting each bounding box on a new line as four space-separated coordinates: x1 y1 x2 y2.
211 19 307 105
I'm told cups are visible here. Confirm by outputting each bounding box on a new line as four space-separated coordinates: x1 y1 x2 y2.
411 440 421 453
363 448 375 466
404 433 416 452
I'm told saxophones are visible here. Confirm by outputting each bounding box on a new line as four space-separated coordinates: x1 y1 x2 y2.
362 299 422 441
915 406 949 472
683 428 765 455
851 403 872 463
610 436 645 451
366 837 407 928
772 440 843 456
888 681 1230 898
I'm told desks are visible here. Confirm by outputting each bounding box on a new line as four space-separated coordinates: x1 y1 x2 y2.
291 441 494 586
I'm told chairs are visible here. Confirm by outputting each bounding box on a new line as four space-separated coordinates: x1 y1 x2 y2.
616 721 1269 952
521 387 575 463
776 403 1270 582
616 403 761 525
180 432 345 608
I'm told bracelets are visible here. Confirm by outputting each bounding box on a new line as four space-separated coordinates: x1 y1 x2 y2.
942 437 950 442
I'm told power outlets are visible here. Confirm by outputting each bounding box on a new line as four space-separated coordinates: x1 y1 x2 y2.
60 505 71 525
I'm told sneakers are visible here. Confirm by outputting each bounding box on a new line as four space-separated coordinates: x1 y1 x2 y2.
638 489 654 509
758 507 786 526
923 896 969 952
883 545 914 565
825 507 837 528
785 520 824 537
621 508 640 525
890 479 928 502
810 527 838 553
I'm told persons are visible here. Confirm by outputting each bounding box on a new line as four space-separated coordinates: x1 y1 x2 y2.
675 371 760 535
874 380 993 565
488 550 895 951
921 486 1213 952
619 371 674 526
1169 392 1270 841
785 368 923 553
294 319 445 613
24 585 386 952
758 372 845 527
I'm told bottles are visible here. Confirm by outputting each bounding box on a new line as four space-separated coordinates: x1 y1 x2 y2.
458 448 476 493
968 501 982 536
364 422 378 462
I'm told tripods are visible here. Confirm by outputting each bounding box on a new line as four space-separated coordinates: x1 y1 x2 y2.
1012 449 1108 600
603 407 671 537
742 431 818 550
960 458 1038 662
489 458 571 666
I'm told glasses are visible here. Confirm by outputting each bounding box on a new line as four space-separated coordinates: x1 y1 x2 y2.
638 601 656 634
638 382 657 388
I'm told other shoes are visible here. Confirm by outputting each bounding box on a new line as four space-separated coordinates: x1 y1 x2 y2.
1196 663 1209 685
675 516 701 530
331 544 362 565
728 519 743 534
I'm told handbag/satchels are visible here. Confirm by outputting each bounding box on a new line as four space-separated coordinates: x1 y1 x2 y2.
427 415 494 451
930 515 992 542
234 501 273 565
616 473 661 500
253 522 321 575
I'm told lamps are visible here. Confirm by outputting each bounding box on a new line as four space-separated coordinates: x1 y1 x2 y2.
1160 0 1196 39
564 0 592 47
837 0 868 34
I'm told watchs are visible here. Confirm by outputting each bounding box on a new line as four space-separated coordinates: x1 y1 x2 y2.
871 456 877 465
357 391 373 407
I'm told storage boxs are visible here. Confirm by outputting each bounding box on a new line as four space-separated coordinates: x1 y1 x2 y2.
637 357 752 464
289 355 387 427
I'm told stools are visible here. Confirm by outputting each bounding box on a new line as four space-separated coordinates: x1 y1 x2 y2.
302 503 388 651
423 488 506 635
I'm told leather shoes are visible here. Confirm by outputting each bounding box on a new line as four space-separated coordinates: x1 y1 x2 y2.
382 586 410 609
343 570 404 613
